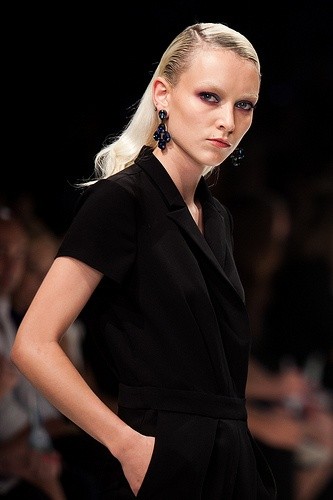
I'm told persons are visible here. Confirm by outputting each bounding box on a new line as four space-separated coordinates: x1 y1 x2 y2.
8 23 268 500
